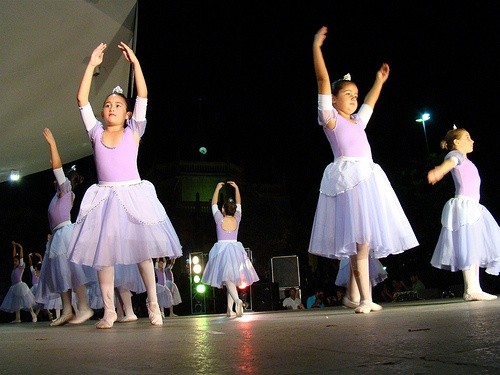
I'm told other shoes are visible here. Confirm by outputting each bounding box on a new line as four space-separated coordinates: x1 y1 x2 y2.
170 314 178 317
344 297 383 311
69 309 94 324
477 290 498 301
463 290 479 301
32 315 38 323
162 313 165 318
120 313 138 322
235 299 244 316
227 310 235 316
355 300 372 314
11 320 22 323
50 313 74 326
146 298 163 325
96 312 118 328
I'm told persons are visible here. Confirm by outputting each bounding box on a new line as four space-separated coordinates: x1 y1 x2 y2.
308 27 421 314
427 129 500 301
36 127 98 327
283 288 343 310
373 273 425 302
0 234 182 323
67 41 183 328
201 182 260 317
